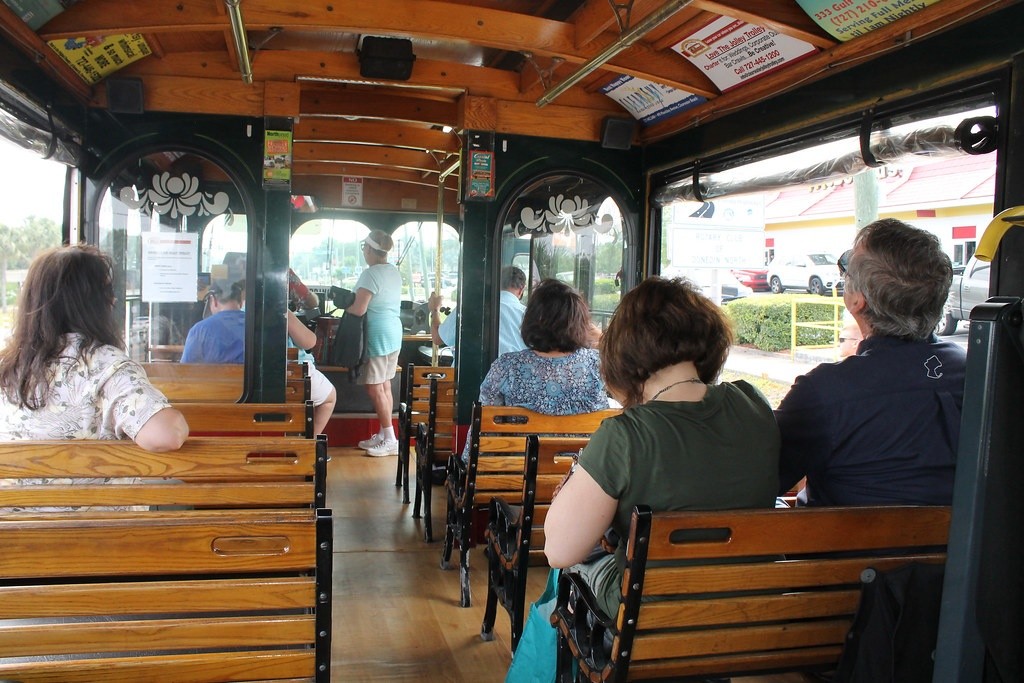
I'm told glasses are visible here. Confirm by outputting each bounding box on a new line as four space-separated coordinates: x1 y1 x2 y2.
838 249 853 281
361 244 367 252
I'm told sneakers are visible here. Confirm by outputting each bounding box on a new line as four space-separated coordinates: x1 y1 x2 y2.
357 434 383 449
366 441 398 456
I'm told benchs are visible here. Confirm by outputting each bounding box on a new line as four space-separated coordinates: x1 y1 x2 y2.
0 346 333 683
394 364 953 683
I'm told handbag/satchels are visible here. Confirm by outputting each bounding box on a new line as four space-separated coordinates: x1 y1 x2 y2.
504 567 561 683
328 309 364 368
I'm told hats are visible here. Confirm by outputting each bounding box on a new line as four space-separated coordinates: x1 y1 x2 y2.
360 235 389 252
202 280 242 320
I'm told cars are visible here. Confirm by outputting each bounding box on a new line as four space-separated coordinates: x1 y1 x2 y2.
555 271 574 282
420 269 458 288
732 267 771 291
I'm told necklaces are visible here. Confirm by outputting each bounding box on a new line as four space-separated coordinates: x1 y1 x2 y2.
650 377 702 401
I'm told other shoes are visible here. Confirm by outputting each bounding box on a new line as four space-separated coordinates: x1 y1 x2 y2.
327 456 331 461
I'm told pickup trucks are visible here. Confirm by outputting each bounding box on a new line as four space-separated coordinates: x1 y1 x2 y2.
933 251 990 336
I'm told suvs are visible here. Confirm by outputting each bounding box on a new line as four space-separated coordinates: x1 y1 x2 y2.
766 250 848 296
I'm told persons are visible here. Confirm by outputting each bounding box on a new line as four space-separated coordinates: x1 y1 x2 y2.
506 273 776 683
775 219 966 506
428 266 529 357
347 229 403 457
179 279 246 363
288 268 333 462
462 277 609 465
0 245 190 453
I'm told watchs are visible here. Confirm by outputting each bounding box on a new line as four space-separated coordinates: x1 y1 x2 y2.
428 308 437 317
